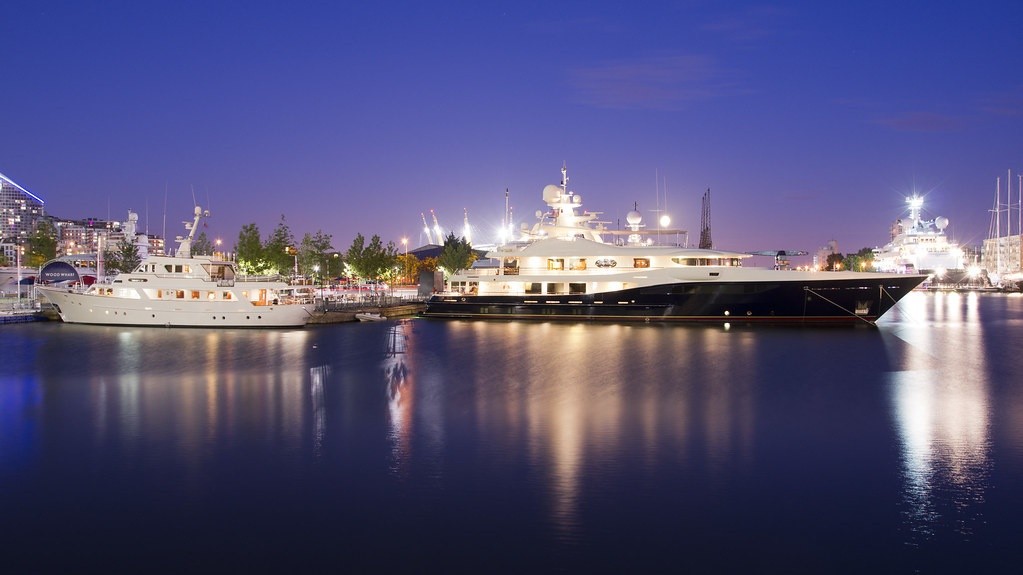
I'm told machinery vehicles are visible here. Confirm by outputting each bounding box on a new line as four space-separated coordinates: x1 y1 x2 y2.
700 188 711 248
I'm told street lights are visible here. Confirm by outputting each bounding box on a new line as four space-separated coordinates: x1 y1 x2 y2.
217 239 221 260
15 238 25 309
403 237 409 286
661 216 671 246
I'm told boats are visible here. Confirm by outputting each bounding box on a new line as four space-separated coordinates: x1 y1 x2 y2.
32 184 316 327
355 312 389 322
42 306 60 321
418 159 929 324
862 170 1023 292
